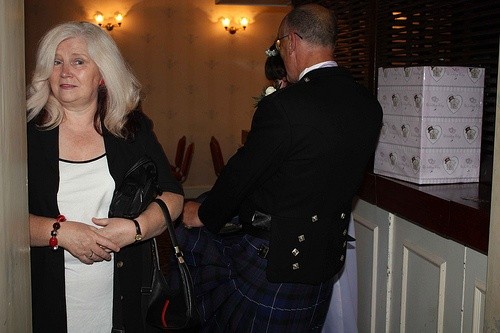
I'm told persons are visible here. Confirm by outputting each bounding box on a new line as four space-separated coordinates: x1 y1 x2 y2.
26 20 186 333
180 2 384 333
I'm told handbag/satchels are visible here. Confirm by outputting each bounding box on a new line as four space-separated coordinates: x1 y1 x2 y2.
141 199 201 332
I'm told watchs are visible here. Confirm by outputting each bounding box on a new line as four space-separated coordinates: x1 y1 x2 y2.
132 219 142 242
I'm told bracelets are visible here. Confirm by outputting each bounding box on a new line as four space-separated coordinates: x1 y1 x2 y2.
49 215 66 250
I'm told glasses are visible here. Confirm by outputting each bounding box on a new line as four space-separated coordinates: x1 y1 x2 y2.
275 32 303 51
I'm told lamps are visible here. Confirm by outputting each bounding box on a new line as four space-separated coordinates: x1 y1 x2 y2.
222 15 248 33
96 10 123 31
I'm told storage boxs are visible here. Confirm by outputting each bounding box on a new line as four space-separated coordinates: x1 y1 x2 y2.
374 65 484 186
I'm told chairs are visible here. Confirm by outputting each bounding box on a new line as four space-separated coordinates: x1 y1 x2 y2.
169 133 224 186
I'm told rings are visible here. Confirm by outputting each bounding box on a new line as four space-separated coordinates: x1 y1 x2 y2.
88 252 94 259
99 245 104 249
